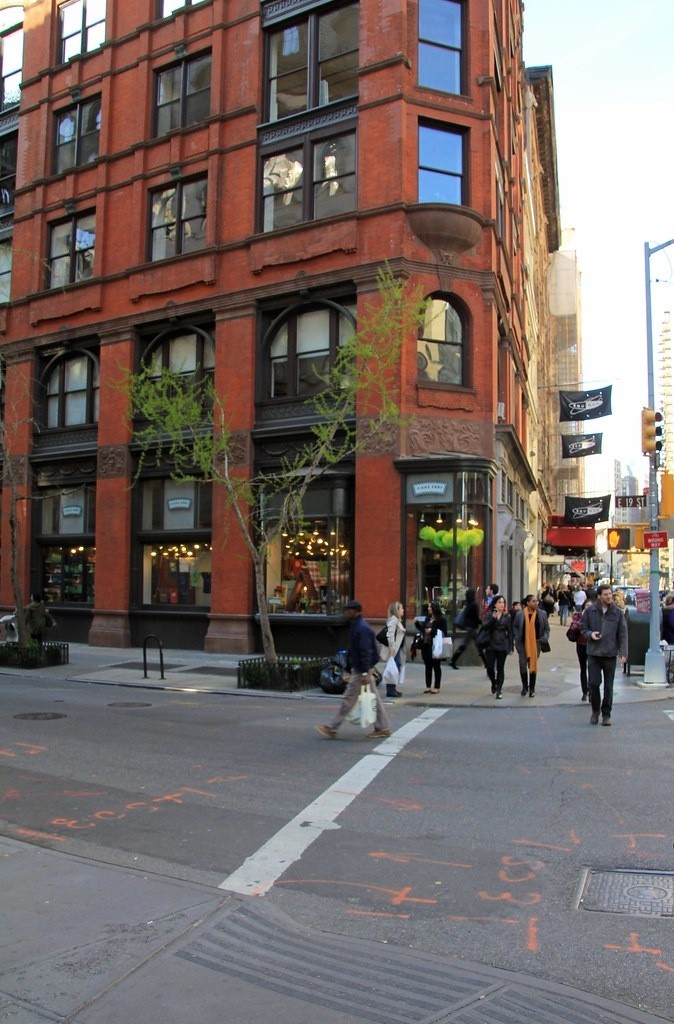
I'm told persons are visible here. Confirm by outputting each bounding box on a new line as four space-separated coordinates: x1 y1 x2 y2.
381 577 674 727
24 592 55 645
314 601 392 739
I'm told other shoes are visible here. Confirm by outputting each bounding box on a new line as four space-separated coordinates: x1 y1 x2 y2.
448 660 459 670
590 711 600 725
602 715 611 726
431 687 440 694
367 728 391 738
496 692 502 699
315 725 337 739
424 687 431 693
491 677 498 694
387 689 401 698
582 692 587 701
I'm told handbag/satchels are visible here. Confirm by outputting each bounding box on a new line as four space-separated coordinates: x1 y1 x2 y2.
432 630 454 659
345 684 378 729
474 612 491 648
412 631 424 650
376 624 405 649
566 613 581 642
381 656 399 685
544 589 555 605
567 600 572 607
453 605 468 629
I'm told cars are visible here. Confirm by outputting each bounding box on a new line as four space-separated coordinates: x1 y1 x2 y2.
612 585 674 605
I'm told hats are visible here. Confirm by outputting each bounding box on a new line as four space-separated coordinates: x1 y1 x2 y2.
342 601 360 608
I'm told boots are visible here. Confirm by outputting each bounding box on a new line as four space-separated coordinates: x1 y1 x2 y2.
521 671 528 696
529 672 536 697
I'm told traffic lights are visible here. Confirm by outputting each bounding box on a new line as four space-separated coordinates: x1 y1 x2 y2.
641 407 663 455
606 527 630 550
